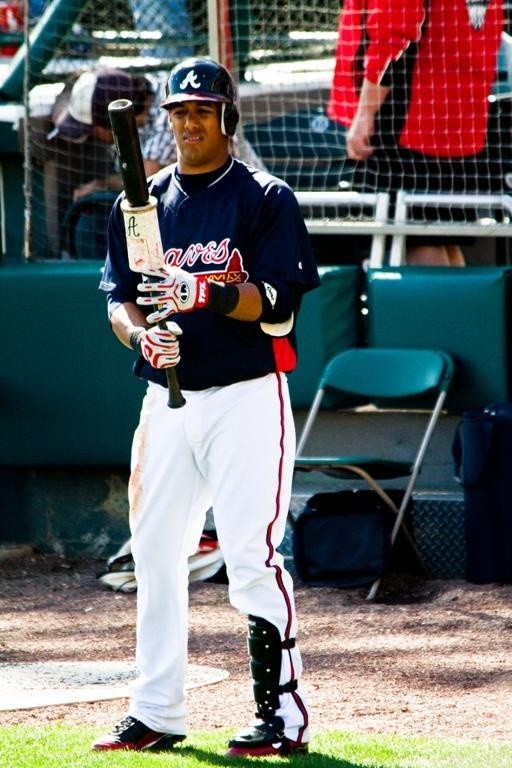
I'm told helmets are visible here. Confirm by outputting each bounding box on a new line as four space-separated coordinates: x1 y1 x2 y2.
159 55 238 113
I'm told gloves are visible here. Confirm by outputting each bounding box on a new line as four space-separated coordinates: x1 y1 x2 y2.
131 318 185 370
136 262 225 326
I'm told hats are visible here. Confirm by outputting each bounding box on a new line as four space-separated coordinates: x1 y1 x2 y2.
52 65 141 143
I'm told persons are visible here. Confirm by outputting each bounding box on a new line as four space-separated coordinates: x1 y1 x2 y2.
90 57 323 758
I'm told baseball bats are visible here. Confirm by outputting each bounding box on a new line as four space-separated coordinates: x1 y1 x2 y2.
108 96 185 408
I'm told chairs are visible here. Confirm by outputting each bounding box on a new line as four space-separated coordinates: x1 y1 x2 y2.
248 106 356 218
282 342 458 609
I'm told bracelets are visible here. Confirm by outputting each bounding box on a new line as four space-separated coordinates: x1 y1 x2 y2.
127 325 144 356
206 276 239 318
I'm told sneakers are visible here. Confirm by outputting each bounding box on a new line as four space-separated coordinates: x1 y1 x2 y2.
226 728 310 761
93 714 186 755
